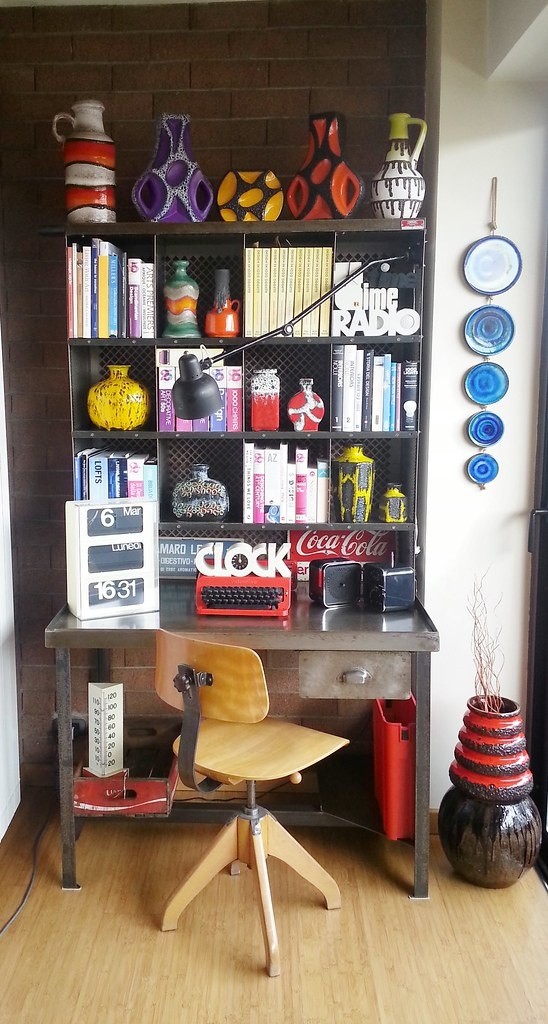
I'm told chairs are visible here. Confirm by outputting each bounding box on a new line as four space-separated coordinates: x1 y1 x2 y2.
156 627 352 979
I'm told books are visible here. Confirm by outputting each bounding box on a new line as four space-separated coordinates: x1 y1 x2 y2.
245 247 332 337
158 366 242 432
332 344 418 432
74 447 157 501
244 442 328 524
67 238 155 340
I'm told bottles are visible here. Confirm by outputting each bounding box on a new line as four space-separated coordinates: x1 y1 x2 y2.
172 464 231 523
85 364 152 432
377 482 409 523
332 442 375 524
162 261 203 338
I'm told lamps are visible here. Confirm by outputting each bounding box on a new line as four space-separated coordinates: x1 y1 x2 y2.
171 241 420 420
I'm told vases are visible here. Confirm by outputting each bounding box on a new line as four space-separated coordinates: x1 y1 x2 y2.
87 364 152 431
378 482 409 523
162 261 202 338
285 111 367 220
128 117 213 223
438 698 542 890
250 369 280 431
215 168 284 223
52 99 116 223
370 112 427 218
287 378 325 432
204 271 241 338
334 443 375 522
171 463 229 522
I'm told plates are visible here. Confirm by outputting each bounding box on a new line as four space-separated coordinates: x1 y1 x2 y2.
464 306 515 357
465 454 499 484
464 362 508 405
467 411 503 448
463 234 523 298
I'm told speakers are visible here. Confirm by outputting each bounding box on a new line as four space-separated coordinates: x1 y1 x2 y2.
309 557 362 608
362 563 416 613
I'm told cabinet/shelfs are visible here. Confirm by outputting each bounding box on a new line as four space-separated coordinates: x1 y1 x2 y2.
44 215 439 897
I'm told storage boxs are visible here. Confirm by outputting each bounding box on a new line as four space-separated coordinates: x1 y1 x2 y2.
73 715 185 818
